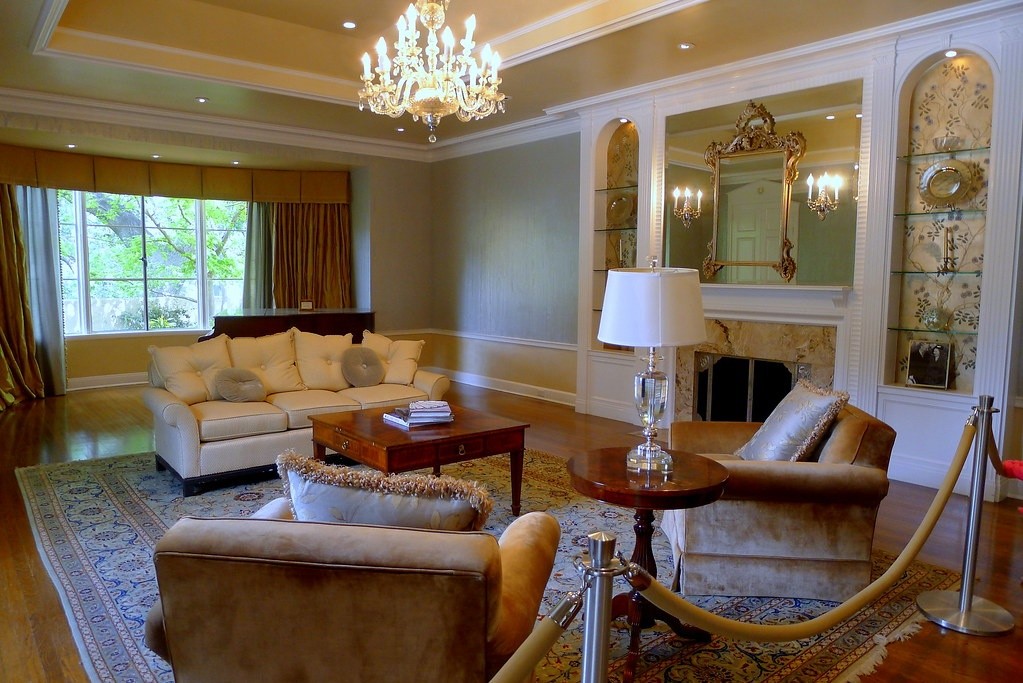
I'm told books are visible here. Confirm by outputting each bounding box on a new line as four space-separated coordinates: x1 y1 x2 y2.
383 401 456 427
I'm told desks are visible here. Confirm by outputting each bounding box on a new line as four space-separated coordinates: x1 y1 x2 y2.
307 402 532 518
564 446 730 683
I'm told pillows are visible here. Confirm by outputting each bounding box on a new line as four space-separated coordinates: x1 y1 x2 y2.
340 347 387 387
215 367 267 403
275 449 493 531
734 378 851 461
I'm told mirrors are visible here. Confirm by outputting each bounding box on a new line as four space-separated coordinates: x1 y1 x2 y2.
661 78 862 290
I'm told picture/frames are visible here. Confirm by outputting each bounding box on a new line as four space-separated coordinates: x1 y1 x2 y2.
905 340 951 391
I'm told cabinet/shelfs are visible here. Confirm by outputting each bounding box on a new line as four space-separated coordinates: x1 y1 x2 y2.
879 50 996 399
591 117 639 353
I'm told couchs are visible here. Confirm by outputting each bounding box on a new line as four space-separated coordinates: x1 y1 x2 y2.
140 329 451 500
142 496 562 683
670 400 898 602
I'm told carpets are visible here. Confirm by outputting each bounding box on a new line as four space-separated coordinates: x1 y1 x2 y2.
13 443 962 683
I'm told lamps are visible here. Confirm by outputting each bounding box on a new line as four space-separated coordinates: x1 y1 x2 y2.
672 187 703 227
805 172 840 221
357 0 506 143
597 255 704 472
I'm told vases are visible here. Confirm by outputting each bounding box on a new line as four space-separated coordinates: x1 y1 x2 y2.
922 306 950 331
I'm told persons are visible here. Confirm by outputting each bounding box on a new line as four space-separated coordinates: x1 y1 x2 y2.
909 342 944 385
